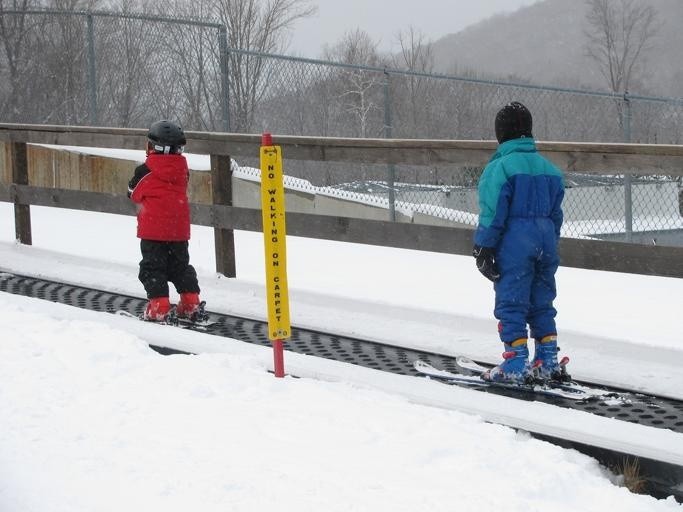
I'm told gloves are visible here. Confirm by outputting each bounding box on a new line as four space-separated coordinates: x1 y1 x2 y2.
473 245 501 283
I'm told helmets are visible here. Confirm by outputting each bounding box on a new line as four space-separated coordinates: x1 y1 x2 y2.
147 120 186 146
494 101 532 144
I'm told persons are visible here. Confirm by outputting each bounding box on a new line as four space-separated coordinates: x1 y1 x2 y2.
471 99 571 387
125 118 207 320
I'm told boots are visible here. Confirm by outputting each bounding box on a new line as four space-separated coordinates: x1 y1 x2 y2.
481 334 561 385
144 292 203 321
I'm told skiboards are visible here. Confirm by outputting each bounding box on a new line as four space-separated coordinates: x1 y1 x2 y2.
115 304 216 333
413 355 618 400
